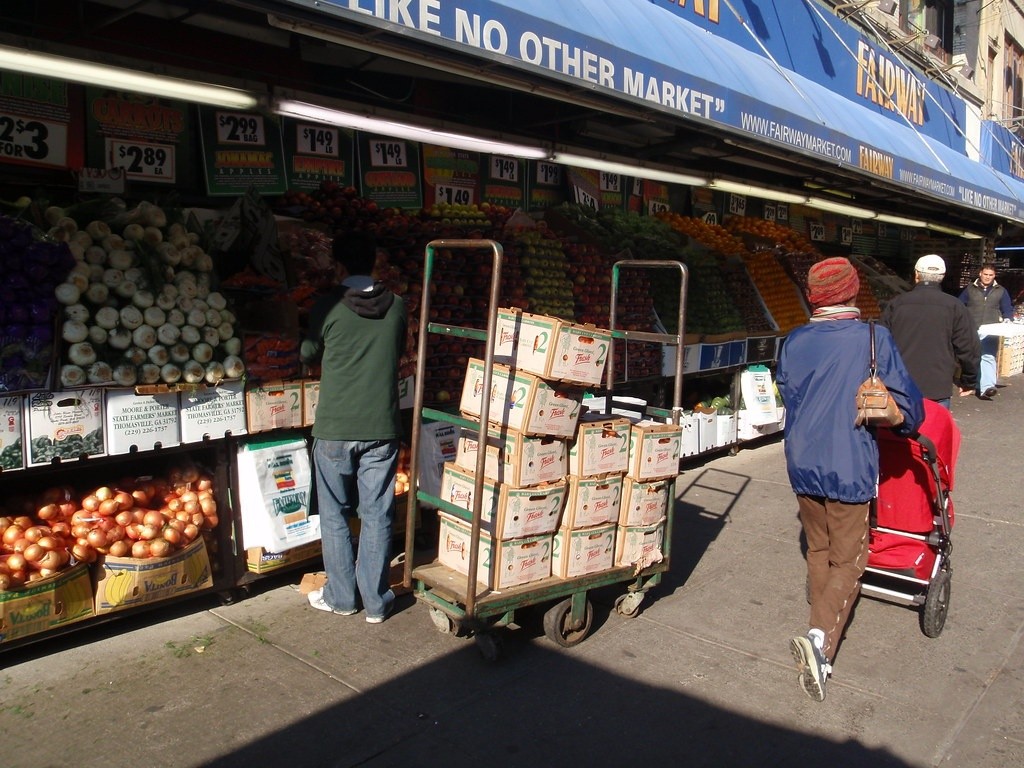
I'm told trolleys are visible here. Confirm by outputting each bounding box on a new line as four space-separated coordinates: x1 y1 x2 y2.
402 240 688 661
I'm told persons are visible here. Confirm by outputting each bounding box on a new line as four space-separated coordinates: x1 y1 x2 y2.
301 231 408 624
879 253 982 411
959 265 1015 400
776 258 925 702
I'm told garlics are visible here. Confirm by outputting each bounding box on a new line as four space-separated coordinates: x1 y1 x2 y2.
44 202 245 385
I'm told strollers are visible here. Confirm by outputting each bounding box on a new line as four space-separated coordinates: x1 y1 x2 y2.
806 397 961 638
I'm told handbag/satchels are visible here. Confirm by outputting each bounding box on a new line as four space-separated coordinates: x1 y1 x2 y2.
852 322 906 428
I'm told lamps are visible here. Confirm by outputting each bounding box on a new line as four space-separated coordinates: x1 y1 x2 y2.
884 29 941 52
927 60 976 79
833 0 897 18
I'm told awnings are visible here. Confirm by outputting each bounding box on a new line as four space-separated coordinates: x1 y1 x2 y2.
249 0 1023 224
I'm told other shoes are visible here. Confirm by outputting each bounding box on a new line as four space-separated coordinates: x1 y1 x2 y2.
981 387 998 399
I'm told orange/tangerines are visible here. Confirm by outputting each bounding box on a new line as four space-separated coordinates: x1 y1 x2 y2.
654 211 881 330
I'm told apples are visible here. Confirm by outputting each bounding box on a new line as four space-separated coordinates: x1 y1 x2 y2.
272 183 747 495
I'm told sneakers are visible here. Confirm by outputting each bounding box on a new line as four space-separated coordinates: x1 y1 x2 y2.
789 629 831 702
307 587 396 624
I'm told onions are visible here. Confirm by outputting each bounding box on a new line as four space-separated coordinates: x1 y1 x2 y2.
0 462 221 588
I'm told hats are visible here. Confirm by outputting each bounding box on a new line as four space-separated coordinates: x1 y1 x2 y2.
914 254 946 275
803 256 859 308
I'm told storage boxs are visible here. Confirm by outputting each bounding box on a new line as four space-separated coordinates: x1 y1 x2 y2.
850 251 914 294
0 304 790 645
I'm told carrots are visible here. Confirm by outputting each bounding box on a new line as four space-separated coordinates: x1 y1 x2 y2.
224 271 320 382
